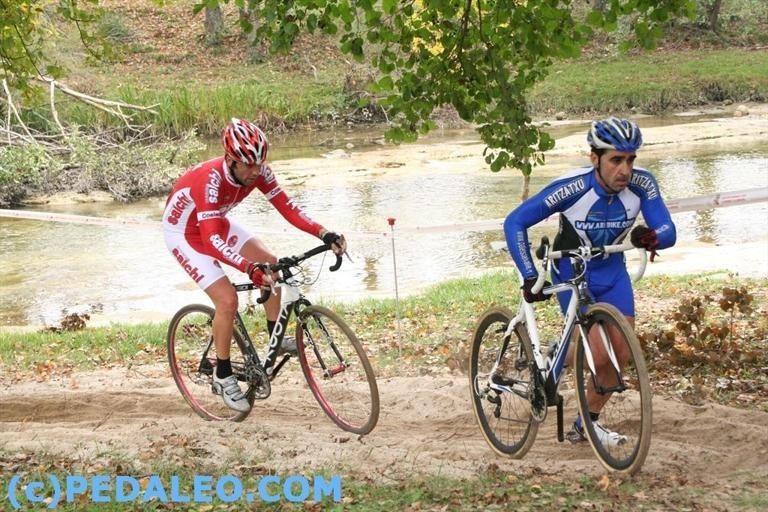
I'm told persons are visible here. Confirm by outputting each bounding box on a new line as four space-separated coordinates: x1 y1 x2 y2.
161 117 347 414
504 115 676 445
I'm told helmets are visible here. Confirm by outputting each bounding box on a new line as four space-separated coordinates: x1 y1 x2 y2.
221 116 268 166
587 116 642 151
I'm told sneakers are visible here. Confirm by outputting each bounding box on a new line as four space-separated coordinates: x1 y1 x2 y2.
566 420 628 448
211 366 251 413
269 333 311 357
545 337 568 390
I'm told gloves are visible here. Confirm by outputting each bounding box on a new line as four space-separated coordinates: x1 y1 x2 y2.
630 222 659 251
522 276 553 303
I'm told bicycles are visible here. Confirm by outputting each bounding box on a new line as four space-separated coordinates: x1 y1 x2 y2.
167 239 381 437
467 235 659 478
